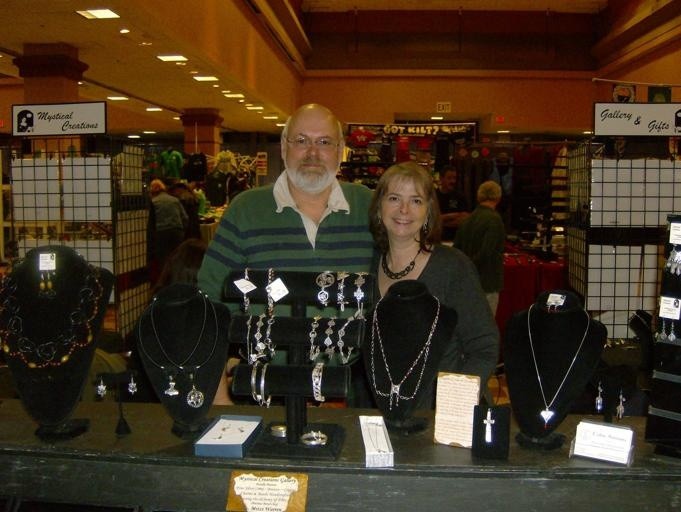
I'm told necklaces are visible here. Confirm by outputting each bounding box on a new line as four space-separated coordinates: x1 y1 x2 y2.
526 303 590 423
370 293 440 409
137 291 207 396
381 247 422 279
366 422 385 453
150 291 219 408
0 262 104 369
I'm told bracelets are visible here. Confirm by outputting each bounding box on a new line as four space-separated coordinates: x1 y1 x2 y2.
235 266 368 447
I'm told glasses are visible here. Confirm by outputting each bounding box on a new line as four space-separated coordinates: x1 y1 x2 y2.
284 135 340 150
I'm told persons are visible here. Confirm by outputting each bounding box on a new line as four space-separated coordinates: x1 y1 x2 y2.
157 146 184 178
368 161 499 408
344 123 411 163
148 179 206 289
436 166 507 292
196 102 376 406
